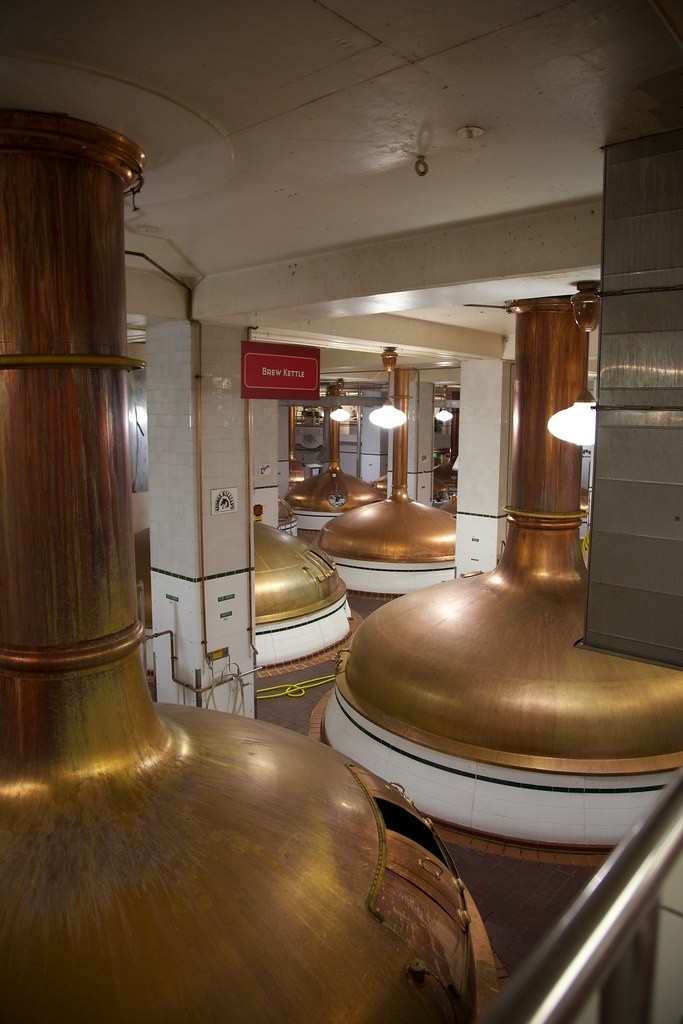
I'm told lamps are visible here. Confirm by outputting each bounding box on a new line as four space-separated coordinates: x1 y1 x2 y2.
546 279 601 448
435 385 453 422
368 347 407 428
330 378 351 422
